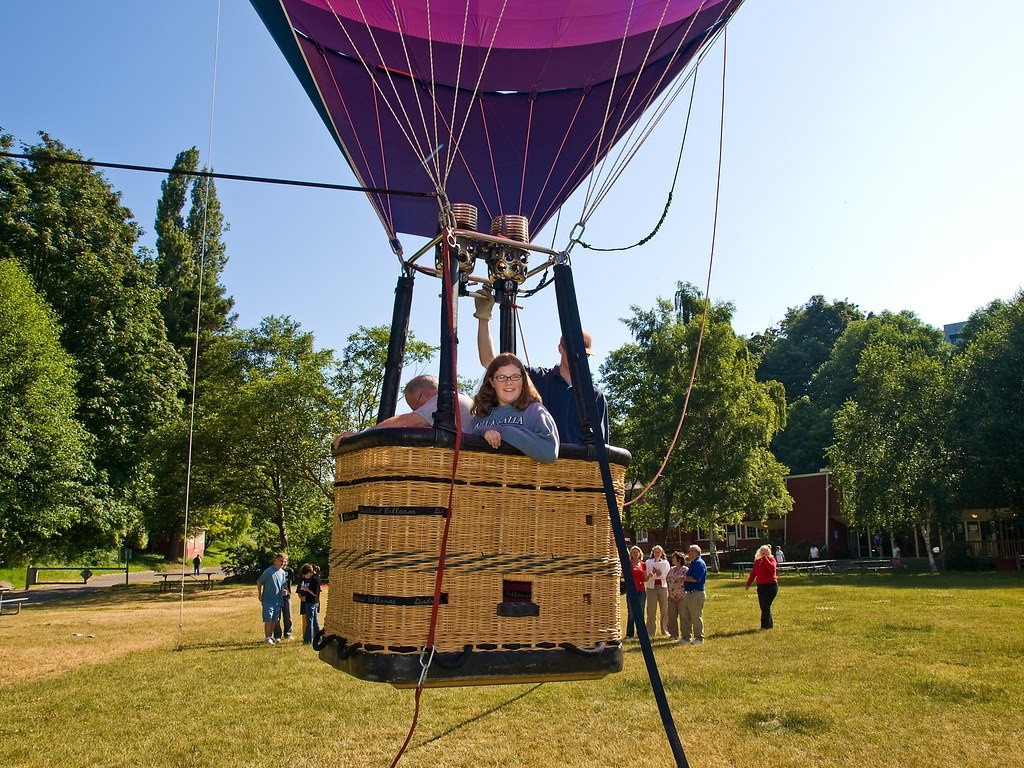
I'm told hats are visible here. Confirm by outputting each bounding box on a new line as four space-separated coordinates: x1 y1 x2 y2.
560 331 595 356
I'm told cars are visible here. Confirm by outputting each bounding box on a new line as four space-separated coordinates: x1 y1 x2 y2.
619 547 630 589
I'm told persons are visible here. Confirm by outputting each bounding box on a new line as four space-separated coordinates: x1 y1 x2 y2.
473 283 610 446
768 544 785 575
333 375 478 449
674 544 707 643
645 545 670 638
665 551 689 640
192 554 200 575
626 544 652 646
746 545 779 631
256 553 321 646
463 353 560 463
892 543 907 570
809 543 819 572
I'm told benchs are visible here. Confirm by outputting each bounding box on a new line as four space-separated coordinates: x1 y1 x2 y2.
0 597 30 616
152 579 220 582
726 570 746 573
848 566 866 570
746 564 830 571
867 566 900 570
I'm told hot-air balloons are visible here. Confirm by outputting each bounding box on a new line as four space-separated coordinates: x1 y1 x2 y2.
250 1 746 689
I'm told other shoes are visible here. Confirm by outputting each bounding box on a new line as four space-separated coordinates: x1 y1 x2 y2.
264 637 275 645
668 636 678 641
275 638 281 642
691 640 703 644
287 636 294 641
676 638 689 642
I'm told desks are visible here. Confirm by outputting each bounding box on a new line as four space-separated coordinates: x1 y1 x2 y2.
847 560 893 572
778 561 815 576
808 558 838 574
153 570 219 593
0 586 11 610
730 561 755 578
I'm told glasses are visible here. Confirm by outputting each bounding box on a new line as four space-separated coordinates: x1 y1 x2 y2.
688 550 692 553
492 373 522 382
